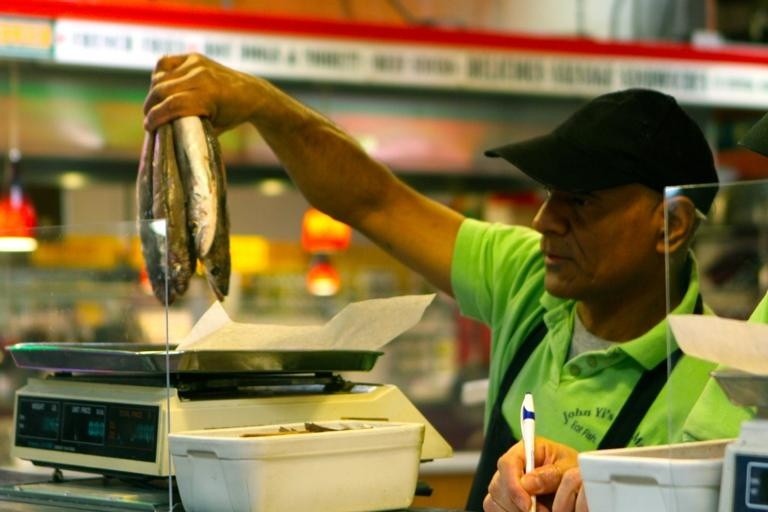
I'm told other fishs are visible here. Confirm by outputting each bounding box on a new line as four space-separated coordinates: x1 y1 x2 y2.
134 115 231 306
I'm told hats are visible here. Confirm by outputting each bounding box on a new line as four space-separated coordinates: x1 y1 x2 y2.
483 86 721 218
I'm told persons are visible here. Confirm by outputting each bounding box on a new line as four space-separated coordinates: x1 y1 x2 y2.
143 52 732 512
481 109 768 512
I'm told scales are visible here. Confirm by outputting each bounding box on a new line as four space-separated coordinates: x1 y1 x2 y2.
4 342 452 495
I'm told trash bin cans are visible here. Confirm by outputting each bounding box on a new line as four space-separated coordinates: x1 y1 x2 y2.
520 392 537 512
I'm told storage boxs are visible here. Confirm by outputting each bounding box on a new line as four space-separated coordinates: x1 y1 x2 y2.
577 438 737 512
169 420 426 512
